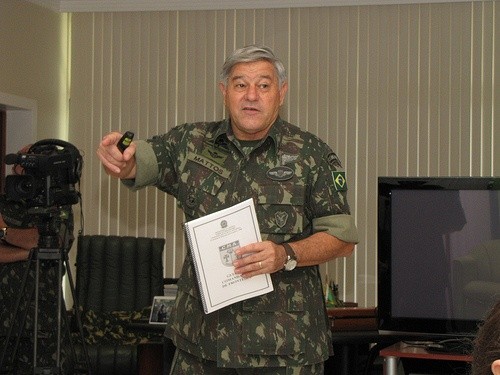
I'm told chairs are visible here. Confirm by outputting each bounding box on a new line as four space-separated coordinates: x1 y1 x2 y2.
62 235 167 375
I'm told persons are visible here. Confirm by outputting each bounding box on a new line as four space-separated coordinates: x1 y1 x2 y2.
0 141 78 375
95 45 359 375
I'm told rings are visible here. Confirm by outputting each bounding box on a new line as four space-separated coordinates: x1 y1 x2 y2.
258 260 262 269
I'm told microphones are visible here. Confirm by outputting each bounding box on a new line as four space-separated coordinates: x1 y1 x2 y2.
4 153 20 165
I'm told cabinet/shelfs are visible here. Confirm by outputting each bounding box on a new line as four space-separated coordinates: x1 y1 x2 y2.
379 341 474 375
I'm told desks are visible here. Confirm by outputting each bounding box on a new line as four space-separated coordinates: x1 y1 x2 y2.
129 307 379 375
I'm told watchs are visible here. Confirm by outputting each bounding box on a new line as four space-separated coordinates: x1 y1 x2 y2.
0 224 8 240
279 241 297 273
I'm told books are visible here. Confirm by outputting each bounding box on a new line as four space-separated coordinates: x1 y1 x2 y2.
183 197 274 313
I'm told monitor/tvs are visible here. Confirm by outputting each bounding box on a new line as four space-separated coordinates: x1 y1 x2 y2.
376 176 500 338
